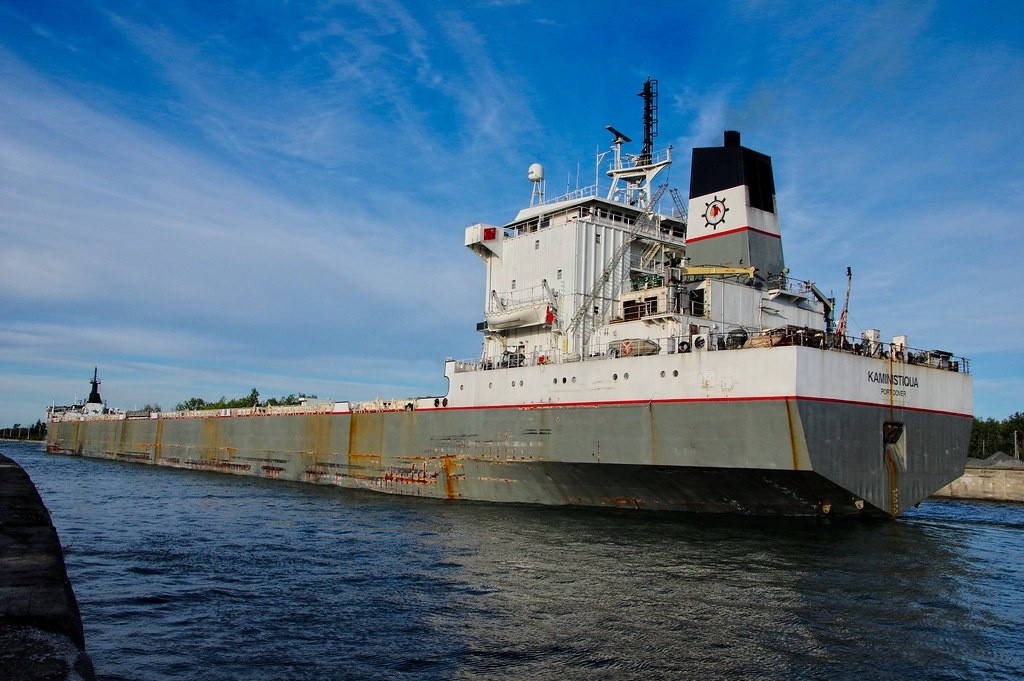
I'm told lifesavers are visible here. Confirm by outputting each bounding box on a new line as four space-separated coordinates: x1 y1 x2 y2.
623 342 633 354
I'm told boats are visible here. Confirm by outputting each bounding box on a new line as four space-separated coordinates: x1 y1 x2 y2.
43 76 976 529
